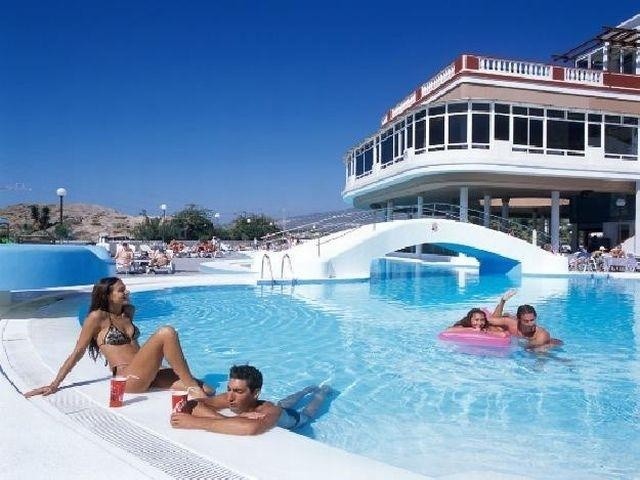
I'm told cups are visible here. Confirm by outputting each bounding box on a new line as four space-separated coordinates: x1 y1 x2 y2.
171 391 188 424
109 377 126 407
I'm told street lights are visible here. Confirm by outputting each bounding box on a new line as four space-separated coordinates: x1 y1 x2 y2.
215 213 223 239
247 219 252 240
160 204 167 243
269 223 274 232
56 187 67 244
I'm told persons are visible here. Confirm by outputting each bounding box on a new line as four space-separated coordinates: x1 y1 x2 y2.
577 245 626 272
23 277 216 398
113 238 218 267
447 308 507 338
489 289 567 369
170 366 333 436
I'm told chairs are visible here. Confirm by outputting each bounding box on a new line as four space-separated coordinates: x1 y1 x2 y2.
96 242 253 273
560 245 640 273
